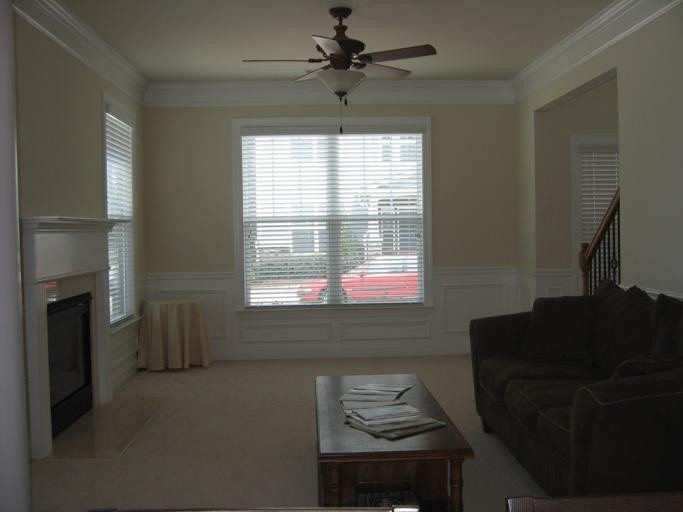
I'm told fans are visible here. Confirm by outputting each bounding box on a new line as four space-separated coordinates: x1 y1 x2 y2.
240 5 437 83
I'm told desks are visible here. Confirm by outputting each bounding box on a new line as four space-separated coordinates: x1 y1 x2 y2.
142 297 201 371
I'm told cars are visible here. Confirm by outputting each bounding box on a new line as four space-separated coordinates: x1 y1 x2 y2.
296 253 420 303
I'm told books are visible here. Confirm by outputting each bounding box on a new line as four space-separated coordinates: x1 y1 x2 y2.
339 384 446 440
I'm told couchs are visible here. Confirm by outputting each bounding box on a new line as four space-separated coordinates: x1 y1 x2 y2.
469 311 683 498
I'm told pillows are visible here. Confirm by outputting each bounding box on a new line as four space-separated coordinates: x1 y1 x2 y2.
522 279 683 360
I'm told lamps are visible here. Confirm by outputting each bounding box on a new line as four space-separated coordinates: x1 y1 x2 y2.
311 66 366 135
